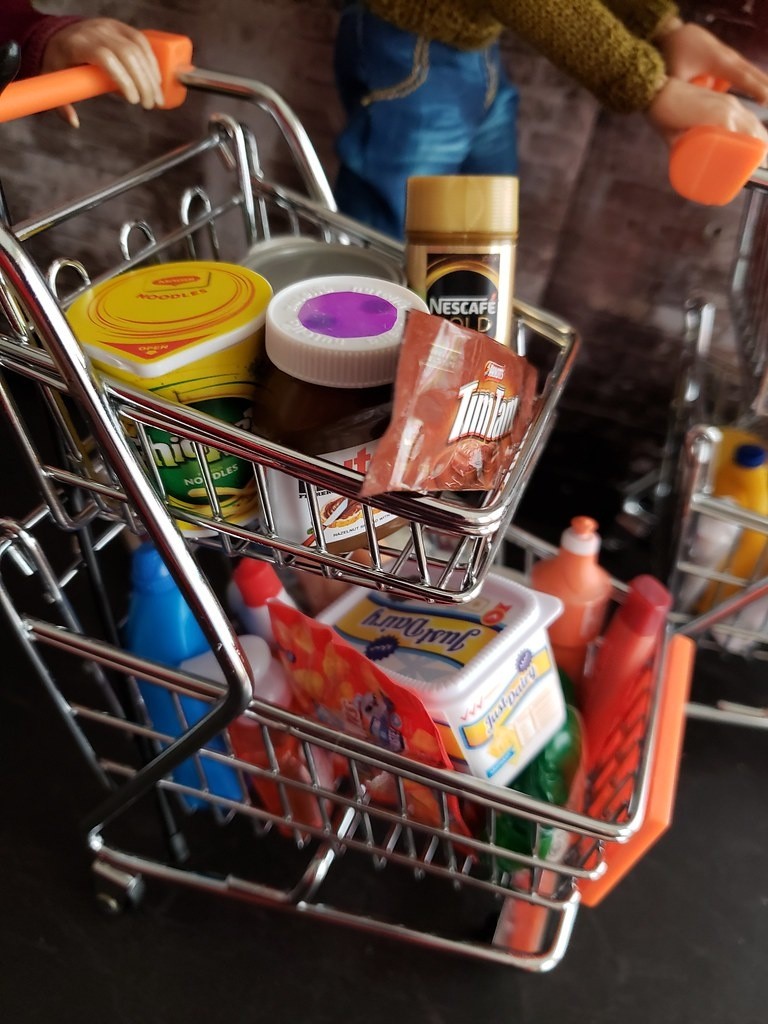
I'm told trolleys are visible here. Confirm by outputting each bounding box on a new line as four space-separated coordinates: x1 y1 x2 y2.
1 21 705 974
642 61 768 725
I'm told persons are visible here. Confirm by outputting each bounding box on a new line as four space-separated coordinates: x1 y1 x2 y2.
0 0 165 129
332 0 768 249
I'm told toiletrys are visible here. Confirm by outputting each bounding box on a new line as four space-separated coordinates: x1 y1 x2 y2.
582 575 676 781
404 170 521 411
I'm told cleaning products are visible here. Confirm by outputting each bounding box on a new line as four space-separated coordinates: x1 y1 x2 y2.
320 538 576 800
125 540 254 811
262 596 482 861
687 443 768 620
234 548 330 719
